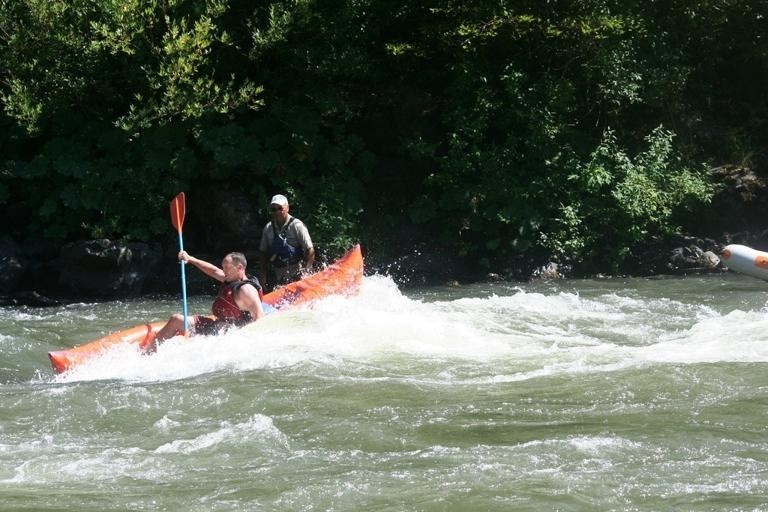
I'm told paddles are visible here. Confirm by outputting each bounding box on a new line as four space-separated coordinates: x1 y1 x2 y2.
170 191 188 341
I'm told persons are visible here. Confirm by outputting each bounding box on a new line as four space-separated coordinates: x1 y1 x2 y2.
258 193 315 293
143 250 265 355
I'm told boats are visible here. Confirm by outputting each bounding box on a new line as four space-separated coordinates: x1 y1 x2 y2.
721 244 768 282
46 243 364 380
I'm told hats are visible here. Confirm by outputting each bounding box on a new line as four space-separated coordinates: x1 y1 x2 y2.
270 194 288 205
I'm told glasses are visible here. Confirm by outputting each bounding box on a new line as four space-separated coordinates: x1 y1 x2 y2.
271 206 283 212
